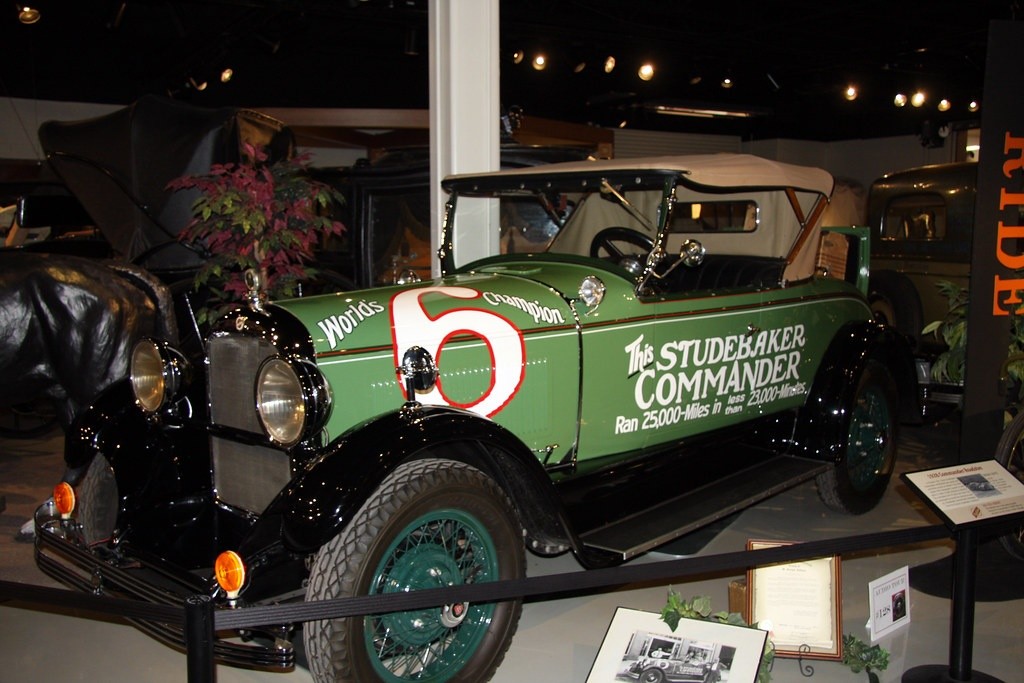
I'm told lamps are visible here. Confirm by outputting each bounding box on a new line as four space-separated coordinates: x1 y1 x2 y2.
15 0 40 24
400 29 421 56
501 42 526 65
254 31 280 54
187 72 207 90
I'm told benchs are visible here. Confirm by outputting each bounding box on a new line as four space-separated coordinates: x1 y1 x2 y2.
602 253 787 301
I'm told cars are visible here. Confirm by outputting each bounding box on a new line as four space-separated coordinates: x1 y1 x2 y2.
10 152 913 683
624 655 723 683
861 161 1024 415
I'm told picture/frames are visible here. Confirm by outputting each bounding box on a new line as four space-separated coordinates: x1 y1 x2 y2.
746 538 843 661
